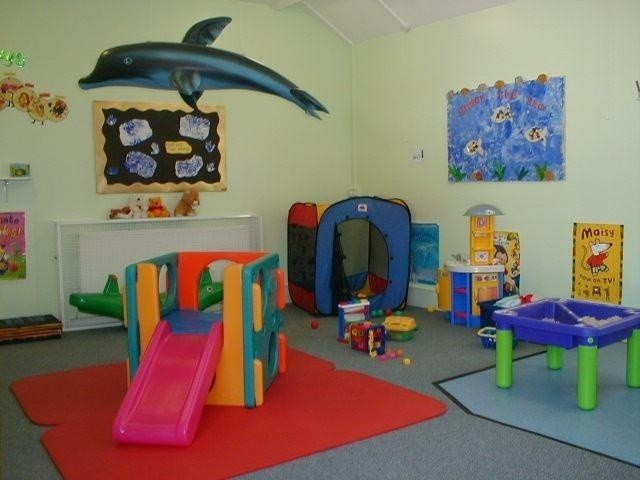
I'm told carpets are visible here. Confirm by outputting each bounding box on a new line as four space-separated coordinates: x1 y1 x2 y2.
7 349 447 479
431 339 640 471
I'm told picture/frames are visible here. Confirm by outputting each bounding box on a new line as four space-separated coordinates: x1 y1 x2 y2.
92 99 228 195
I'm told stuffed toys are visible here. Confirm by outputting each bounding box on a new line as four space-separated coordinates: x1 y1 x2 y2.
108 206 134 219
127 191 148 219
175 190 200 218
147 197 170 219
